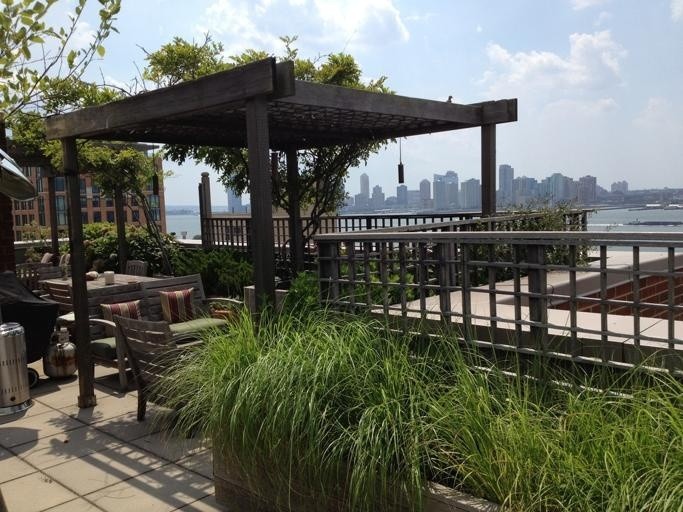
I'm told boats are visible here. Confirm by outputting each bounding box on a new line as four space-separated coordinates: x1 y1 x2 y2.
664 203 683 210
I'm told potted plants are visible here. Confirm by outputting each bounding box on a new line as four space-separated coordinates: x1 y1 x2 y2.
138 283 554 512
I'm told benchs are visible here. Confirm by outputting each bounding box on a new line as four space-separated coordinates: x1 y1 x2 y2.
16 262 52 290
79 273 245 391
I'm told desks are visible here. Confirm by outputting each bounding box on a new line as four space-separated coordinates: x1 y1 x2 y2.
39 274 161 297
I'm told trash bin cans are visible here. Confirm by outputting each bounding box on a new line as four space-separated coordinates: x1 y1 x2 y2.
0 322 34 416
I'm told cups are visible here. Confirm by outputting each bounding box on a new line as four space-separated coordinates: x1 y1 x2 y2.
104 271 114 285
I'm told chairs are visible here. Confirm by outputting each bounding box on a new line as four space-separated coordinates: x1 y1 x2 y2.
47 284 74 331
113 313 206 439
126 259 147 276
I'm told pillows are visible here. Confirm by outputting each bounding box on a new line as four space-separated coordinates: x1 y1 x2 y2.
100 287 195 327
